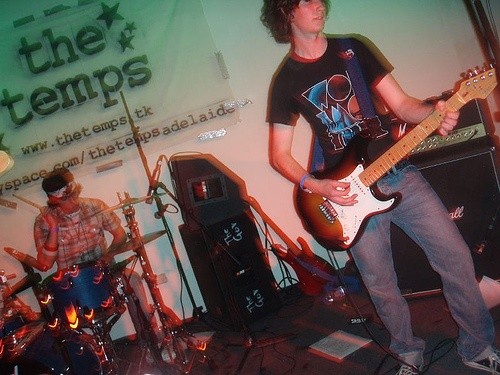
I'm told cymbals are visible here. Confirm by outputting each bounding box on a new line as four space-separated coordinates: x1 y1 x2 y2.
81 192 168 220
98 229 167 257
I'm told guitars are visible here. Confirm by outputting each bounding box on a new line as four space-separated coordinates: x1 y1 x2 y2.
247 196 339 299
293 61 498 252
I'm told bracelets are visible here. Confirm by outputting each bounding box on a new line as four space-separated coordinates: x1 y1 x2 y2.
50 227 59 232
300 174 315 193
44 242 58 251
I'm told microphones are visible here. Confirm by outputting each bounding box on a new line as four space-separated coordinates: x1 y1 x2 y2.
146 155 163 204
4 247 48 273
154 204 168 219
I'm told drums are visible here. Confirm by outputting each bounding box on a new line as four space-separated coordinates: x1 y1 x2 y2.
39 260 122 329
0 321 108 375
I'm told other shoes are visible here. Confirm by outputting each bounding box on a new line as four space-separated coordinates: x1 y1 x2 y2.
145 346 177 361
396 364 424 375
463 350 500 375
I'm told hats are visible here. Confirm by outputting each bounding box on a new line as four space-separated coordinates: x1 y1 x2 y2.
43 168 81 205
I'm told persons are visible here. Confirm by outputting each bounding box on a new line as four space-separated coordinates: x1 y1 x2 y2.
34 167 127 375
260 0 500 375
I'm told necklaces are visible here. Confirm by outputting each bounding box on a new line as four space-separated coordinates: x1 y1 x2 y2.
64 215 81 240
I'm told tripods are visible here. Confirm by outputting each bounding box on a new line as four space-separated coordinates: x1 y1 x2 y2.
158 182 298 375
114 204 219 375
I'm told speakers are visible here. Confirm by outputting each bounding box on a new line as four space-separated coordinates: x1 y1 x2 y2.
390 134 500 299
170 152 284 333
